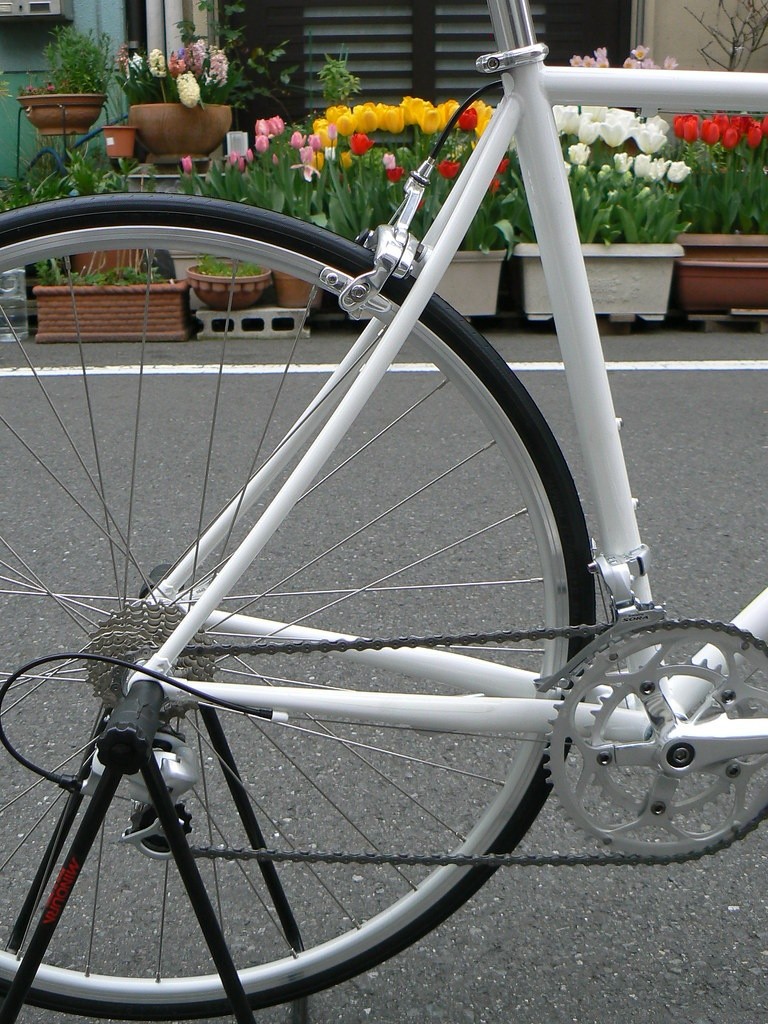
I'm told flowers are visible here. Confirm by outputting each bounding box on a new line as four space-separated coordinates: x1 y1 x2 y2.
176 147 255 206
16 25 116 95
255 47 768 253
113 39 243 111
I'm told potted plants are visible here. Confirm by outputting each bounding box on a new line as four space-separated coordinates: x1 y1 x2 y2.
186 253 273 311
31 267 195 342
59 148 155 271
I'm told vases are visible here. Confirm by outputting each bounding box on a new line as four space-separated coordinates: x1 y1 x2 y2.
15 95 108 136
272 268 322 307
129 104 232 159
514 241 683 320
409 248 506 322
101 126 137 157
673 233 768 312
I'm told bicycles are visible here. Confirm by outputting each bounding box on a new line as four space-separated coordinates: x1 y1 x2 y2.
0 0 768 1024
